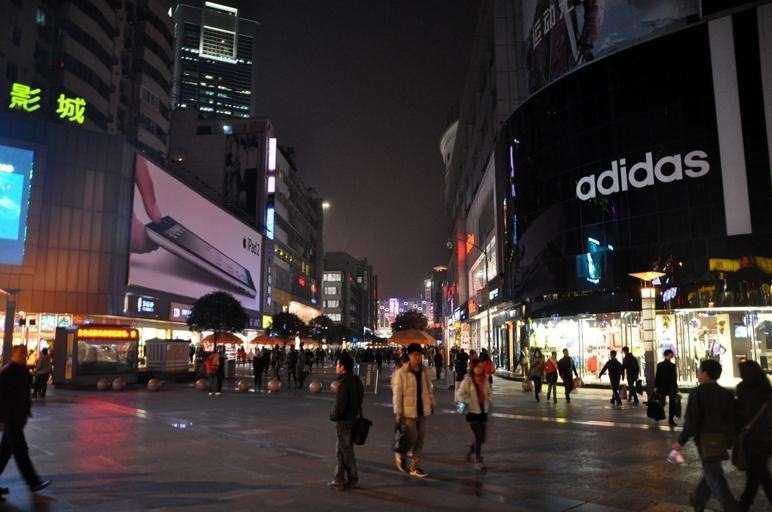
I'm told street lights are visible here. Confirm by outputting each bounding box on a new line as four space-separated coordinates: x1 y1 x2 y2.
447 236 491 358
0 287 25 368
627 270 666 403
19 318 36 349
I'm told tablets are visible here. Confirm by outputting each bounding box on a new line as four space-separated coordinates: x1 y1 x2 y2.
144 214 257 298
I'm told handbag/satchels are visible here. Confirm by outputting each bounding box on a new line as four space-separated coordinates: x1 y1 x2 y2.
674 396 681 417
546 373 556 384
455 401 467 414
643 393 665 420
636 380 643 395
352 416 372 445
731 438 749 471
393 423 410 454
700 432 729 460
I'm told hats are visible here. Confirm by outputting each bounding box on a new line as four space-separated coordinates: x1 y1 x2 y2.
407 343 424 353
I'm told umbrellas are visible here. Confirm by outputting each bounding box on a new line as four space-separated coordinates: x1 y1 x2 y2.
200 331 242 344
249 335 283 346
387 327 436 345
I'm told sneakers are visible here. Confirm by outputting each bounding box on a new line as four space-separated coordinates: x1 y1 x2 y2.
0 486 10 494
409 468 427 478
396 461 406 473
474 461 486 472
30 481 50 492
328 481 342 490
345 478 359 488
669 420 676 427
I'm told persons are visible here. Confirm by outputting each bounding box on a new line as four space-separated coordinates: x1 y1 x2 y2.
129 156 164 255
349 346 410 385
518 346 578 404
452 357 493 471
0 343 52 497
192 344 341 396
32 348 51 400
672 360 737 512
599 348 678 430
422 346 496 402
731 359 772 512
327 351 365 491
388 341 436 478
